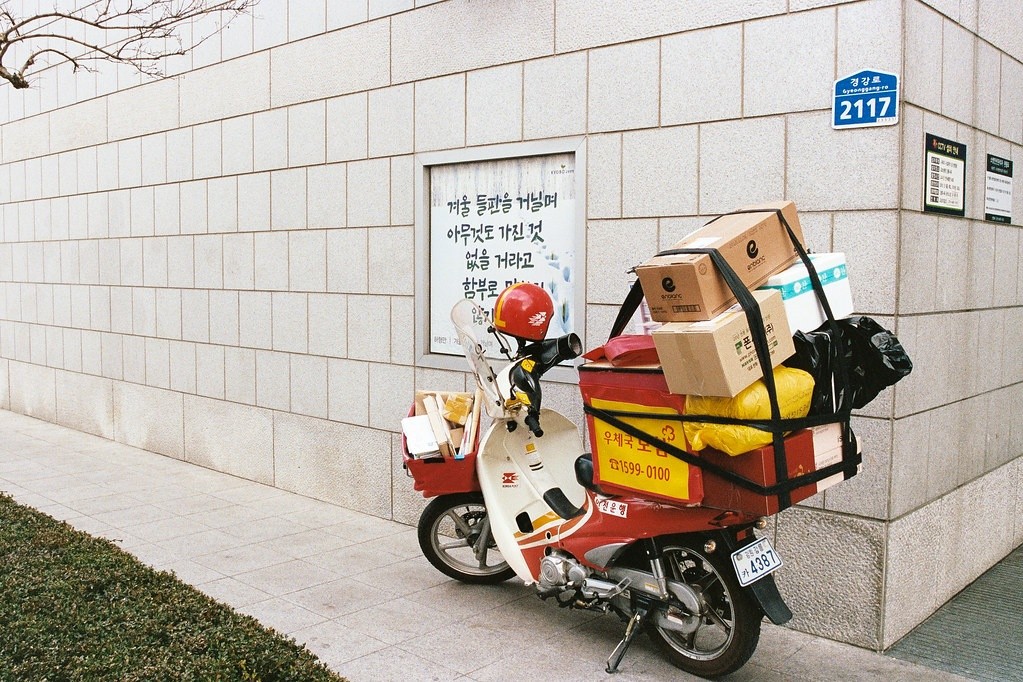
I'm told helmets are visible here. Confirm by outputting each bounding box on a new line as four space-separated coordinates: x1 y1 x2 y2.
494 282 554 341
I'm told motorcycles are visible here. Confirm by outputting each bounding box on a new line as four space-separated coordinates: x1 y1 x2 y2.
403 298 792 679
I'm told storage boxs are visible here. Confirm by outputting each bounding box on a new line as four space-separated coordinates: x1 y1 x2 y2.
577 201 861 517
414 391 456 459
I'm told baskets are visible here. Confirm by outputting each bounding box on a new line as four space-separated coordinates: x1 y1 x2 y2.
402 402 480 497
